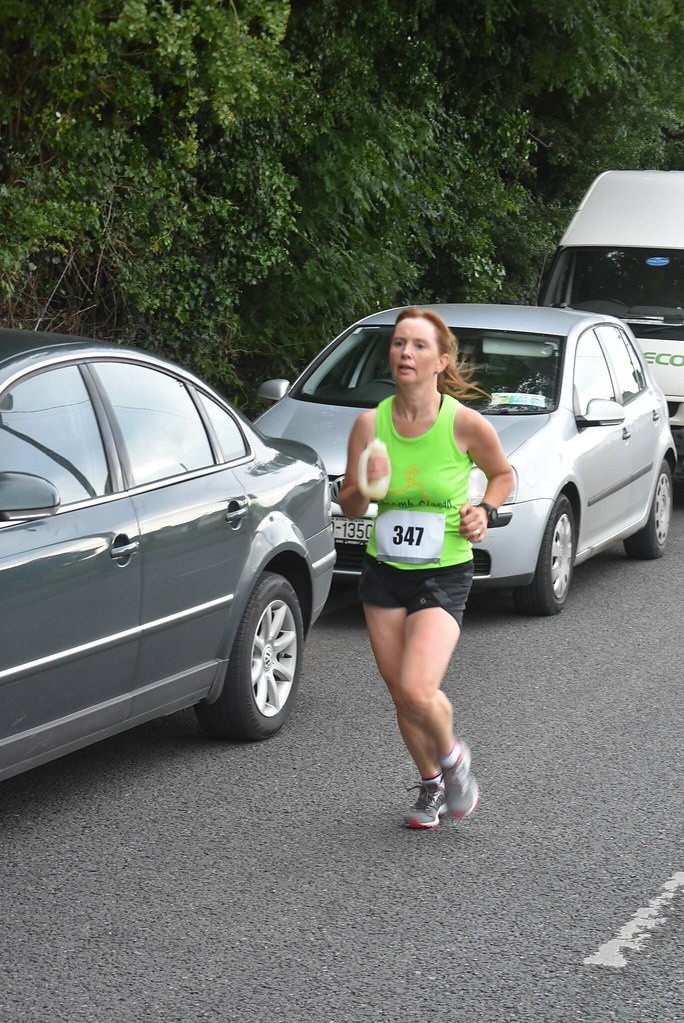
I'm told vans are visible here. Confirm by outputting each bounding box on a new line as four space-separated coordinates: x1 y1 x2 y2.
535 170 684 491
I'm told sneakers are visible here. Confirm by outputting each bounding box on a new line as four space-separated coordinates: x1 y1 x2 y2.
402 779 450 829
441 743 479 816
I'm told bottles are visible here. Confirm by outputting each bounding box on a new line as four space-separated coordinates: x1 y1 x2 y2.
355 438 392 502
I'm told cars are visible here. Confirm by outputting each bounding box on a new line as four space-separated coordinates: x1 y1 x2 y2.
252 304 676 615
0 328 336 784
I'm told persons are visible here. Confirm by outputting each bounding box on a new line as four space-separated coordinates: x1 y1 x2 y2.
340 305 513 832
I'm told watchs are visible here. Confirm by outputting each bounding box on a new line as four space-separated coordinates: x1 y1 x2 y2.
477 503 498 526
